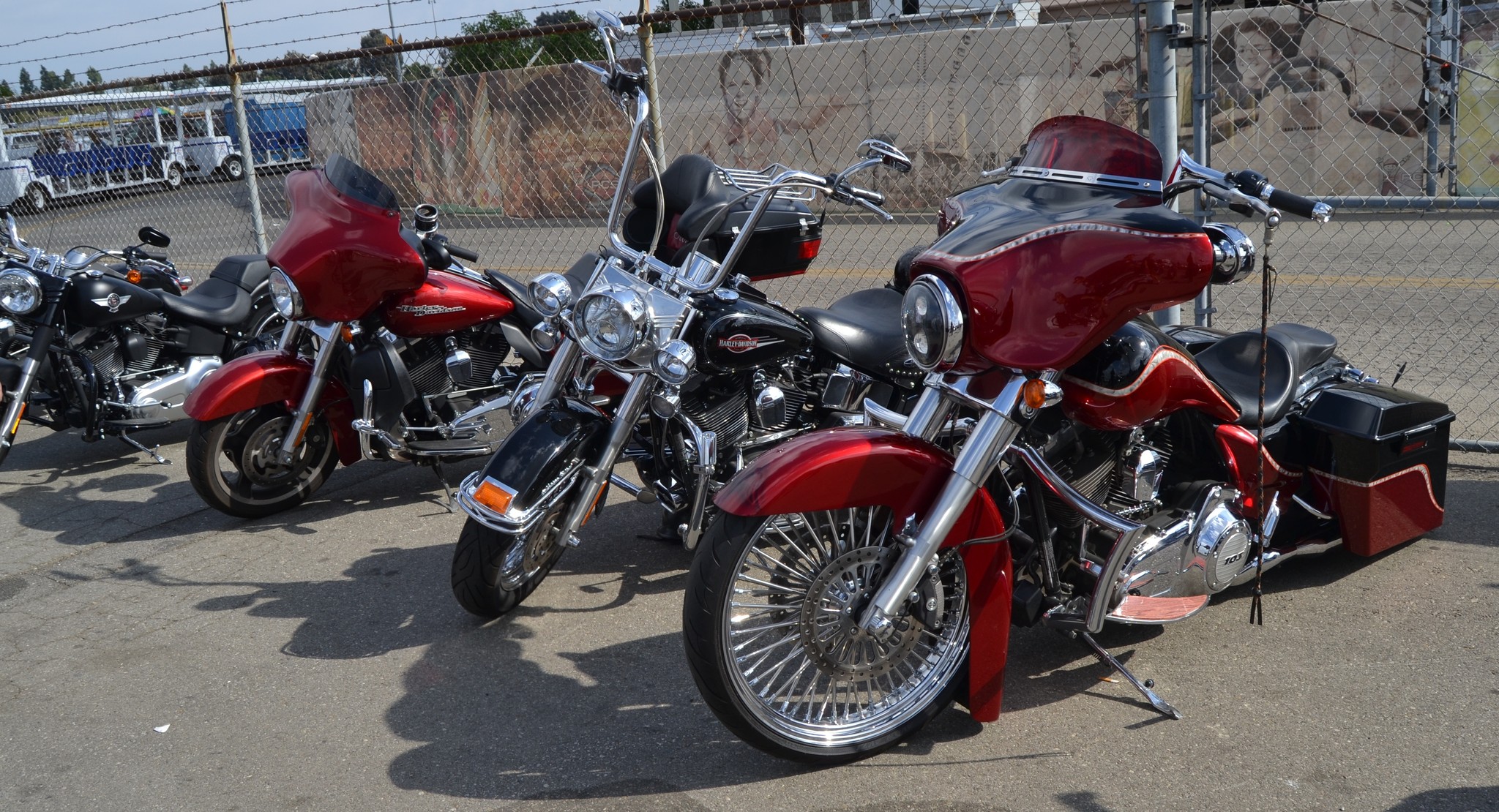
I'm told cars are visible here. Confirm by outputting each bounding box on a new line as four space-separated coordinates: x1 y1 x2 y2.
0 103 226 160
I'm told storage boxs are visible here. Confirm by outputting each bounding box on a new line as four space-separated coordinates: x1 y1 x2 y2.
1300 384 1457 559
717 177 823 283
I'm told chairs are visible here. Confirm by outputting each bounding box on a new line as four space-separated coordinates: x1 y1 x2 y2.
621 152 729 269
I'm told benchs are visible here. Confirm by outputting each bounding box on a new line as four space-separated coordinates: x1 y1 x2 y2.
27 143 154 177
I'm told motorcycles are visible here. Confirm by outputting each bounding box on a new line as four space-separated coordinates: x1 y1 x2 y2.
445 21 929 623
678 115 1455 772
168 151 816 522
1 203 317 477
